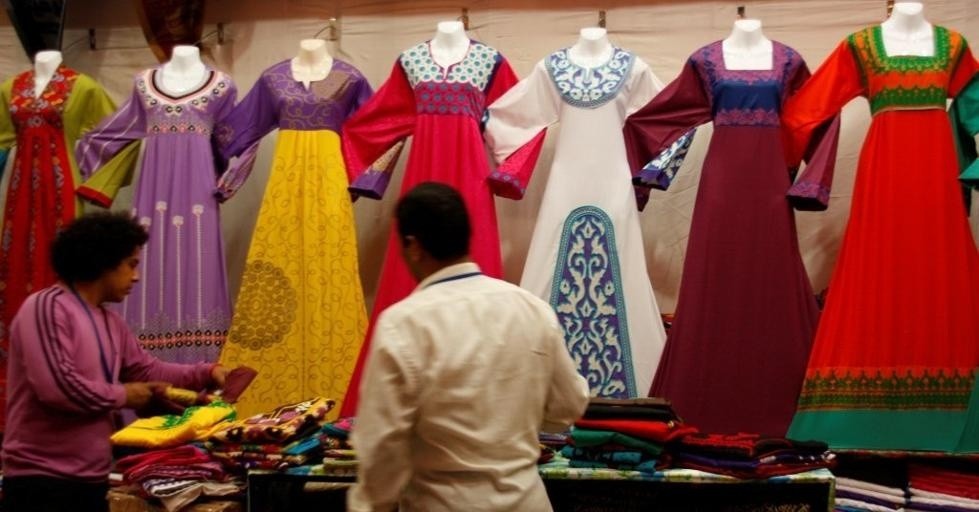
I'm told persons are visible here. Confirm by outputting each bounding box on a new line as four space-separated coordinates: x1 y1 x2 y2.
781 0 979 462
478 24 697 401
1 45 143 376
0 210 229 510
340 181 590 511
72 42 263 369
333 20 550 428
211 37 406 428
621 14 842 439
